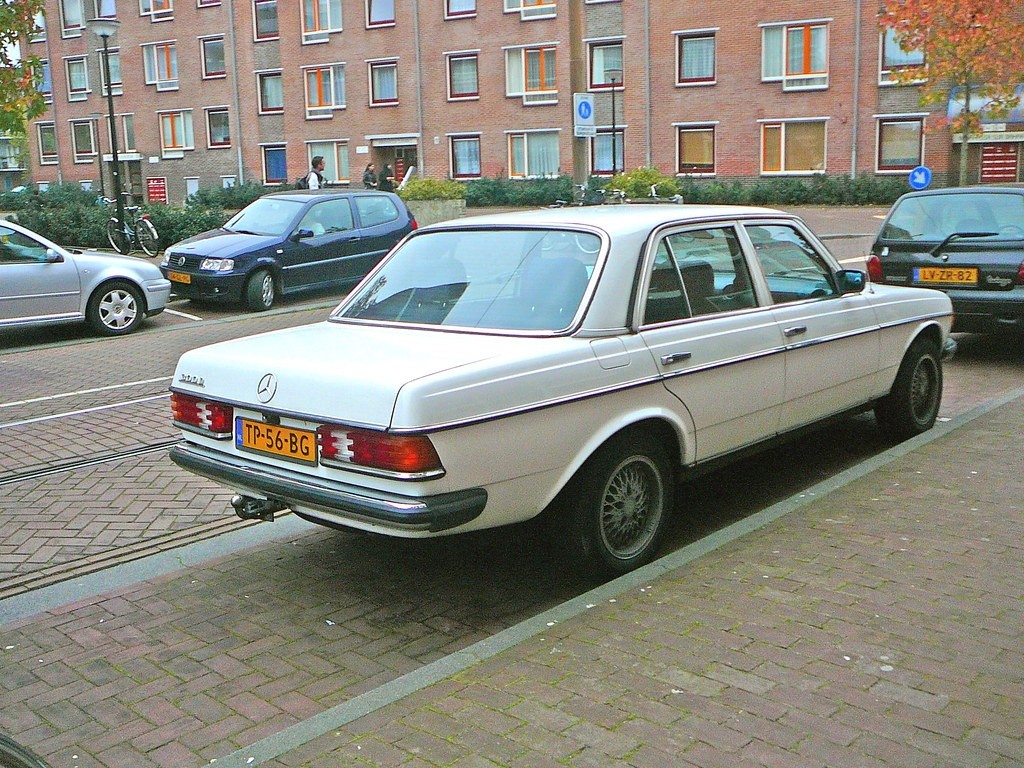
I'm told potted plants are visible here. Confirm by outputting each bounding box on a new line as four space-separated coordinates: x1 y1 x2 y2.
390 175 468 228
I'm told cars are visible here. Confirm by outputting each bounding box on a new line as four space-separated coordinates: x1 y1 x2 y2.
0 219 171 337
159 188 420 313
169 203 958 581
865 183 1024 335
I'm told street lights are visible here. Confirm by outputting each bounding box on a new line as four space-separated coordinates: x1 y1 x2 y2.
85 17 127 257
604 67 624 178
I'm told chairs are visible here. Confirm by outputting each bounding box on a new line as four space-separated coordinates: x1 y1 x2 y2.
654 259 720 317
954 218 983 233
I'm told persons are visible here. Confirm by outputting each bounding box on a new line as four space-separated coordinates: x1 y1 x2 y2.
379 162 395 190
298 211 325 235
363 163 378 189
306 156 326 189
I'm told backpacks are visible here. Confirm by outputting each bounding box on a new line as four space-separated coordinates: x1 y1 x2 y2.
298 171 314 189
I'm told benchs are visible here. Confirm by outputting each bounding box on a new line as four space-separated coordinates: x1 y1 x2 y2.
379 256 588 330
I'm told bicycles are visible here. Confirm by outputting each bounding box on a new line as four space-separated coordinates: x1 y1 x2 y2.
548 182 684 208
96 192 160 259
